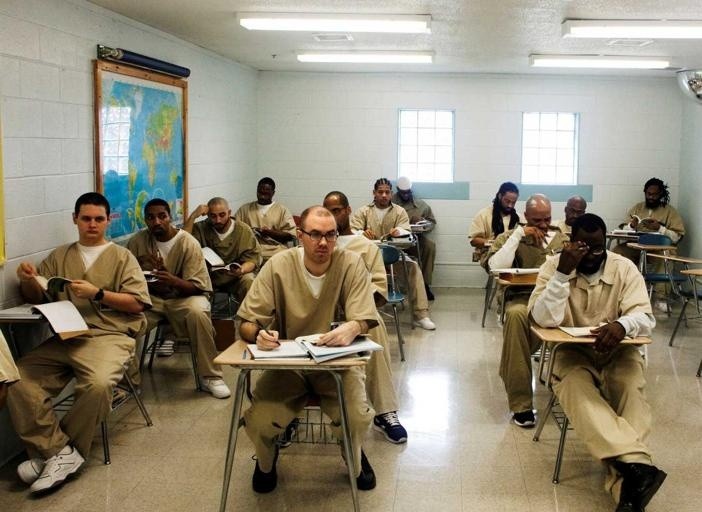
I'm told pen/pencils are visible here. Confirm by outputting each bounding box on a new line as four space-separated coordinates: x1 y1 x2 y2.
629 214 633 219
156 248 160 257
256 319 281 347
556 244 587 254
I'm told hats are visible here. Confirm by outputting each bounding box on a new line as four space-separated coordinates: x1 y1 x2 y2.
397 176 411 190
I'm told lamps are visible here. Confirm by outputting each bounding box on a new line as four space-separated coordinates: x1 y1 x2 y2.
528 17 702 71
233 13 436 66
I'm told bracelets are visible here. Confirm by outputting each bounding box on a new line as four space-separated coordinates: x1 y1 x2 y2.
92 288 104 302
254 329 260 340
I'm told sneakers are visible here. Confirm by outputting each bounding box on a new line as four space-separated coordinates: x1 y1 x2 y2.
497 312 505 327
30 447 84 499
356 447 376 490
252 444 279 493
156 341 173 357
373 411 407 443
275 416 298 448
616 463 667 512
413 317 436 330
513 410 535 428
201 379 230 399
425 284 434 301
655 303 672 313
17 458 45 485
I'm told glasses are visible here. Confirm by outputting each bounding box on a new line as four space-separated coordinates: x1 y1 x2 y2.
302 229 339 242
583 247 604 256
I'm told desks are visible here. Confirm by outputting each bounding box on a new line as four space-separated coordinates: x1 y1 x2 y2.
2 216 701 368
528 324 652 484
0 301 152 465
213 339 372 510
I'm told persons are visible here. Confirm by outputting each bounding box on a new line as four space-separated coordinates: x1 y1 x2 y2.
390 178 437 300
481 193 572 429
350 178 436 330
526 213 667 512
126 197 231 401
468 181 520 273
554 195 588 239
611 177 686 314
183 197 261 304
6 193 154 498
235 204 382 492
234 177 297 260
277 190 409 447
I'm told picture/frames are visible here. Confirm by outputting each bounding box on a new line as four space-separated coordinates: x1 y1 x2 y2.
94 58 189 243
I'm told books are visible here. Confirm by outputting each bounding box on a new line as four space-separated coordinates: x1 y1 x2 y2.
200 248 241 272
612 228 660 237
246 332 327 359
631 214 652 226
490 266 542 276
392 236 413 244
0 302 89 342
307 336 385 365
557 322 633 340
31 271 71 292
368 232 391 245
